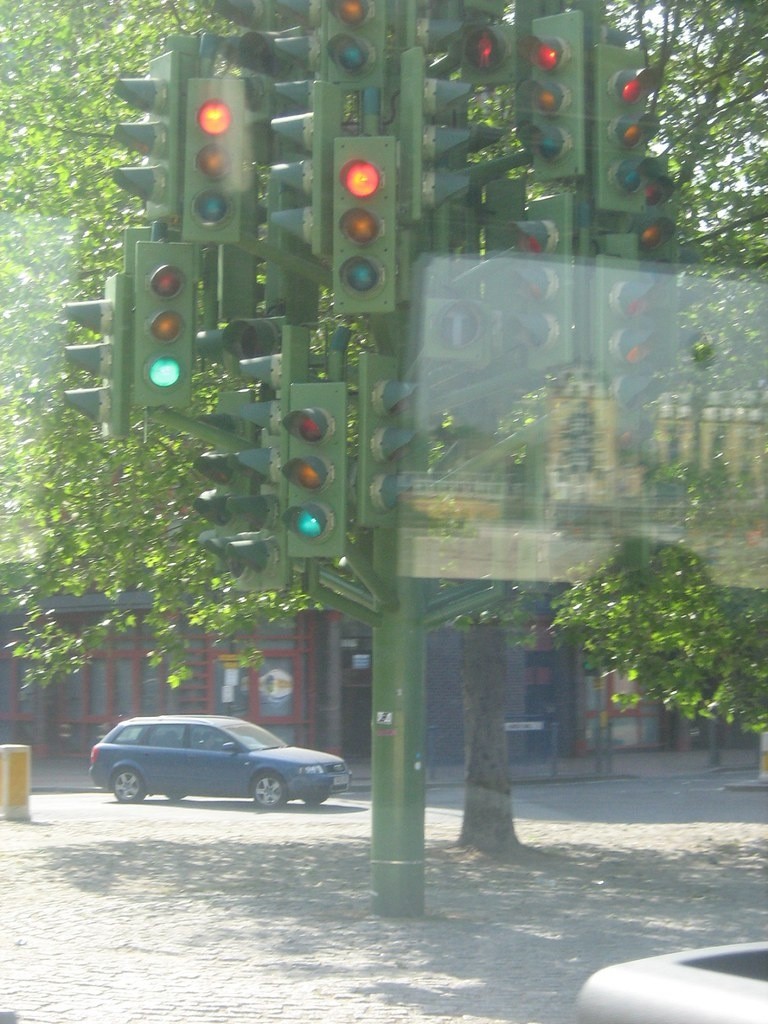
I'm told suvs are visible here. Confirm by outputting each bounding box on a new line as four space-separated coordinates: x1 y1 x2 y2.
86 716 351 809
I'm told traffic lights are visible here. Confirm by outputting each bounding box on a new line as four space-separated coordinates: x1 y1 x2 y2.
521 10 595 181
61 273 132 439
213 3 272 127
333 139 399 309
406 1 467 52
471 29 517 82
195 389 286 596
506 194 577 373
237 326 307 483
587 42 657 211
181 78 245 248
658 223 703 359
623 157 677 311
279 383 351 559
132 240 195 407
356 354 425 525
265 80 328 263
110 51 181 227
321 1 393 121
400 50 473 225
593 247 657 412
